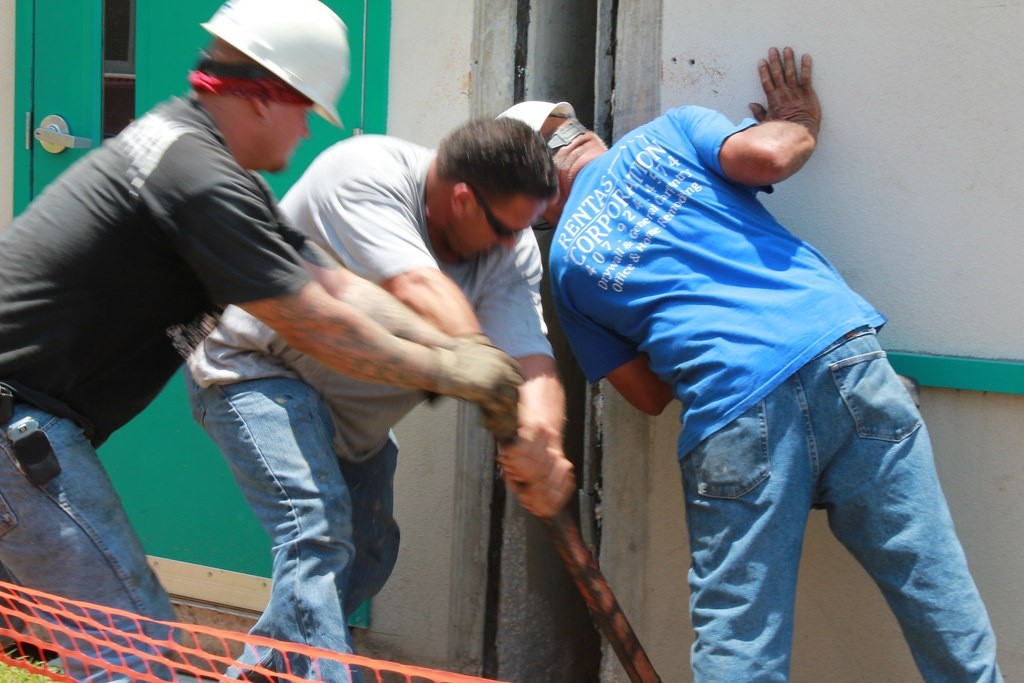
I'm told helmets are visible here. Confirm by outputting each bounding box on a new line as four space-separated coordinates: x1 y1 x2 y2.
495 101 577 134
199 0 350 126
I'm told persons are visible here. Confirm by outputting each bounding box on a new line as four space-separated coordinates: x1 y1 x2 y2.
183 119 574 683
497 47 1004 683
0 1 526 683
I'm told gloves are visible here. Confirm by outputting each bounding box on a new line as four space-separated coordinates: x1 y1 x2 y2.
452 333 520 443
432 344 524 416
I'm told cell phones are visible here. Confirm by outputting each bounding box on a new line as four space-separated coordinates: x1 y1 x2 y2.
6 416 41 445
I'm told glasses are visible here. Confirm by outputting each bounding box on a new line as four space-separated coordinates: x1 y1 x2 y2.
472 186 519 236
